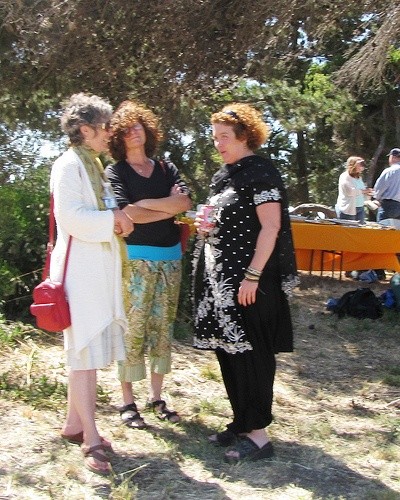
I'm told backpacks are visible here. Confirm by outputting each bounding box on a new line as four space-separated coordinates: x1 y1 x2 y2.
334 287 384 319
376 286 400 313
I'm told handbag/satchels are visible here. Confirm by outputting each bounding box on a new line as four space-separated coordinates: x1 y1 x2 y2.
29 278 71 332
358 270 378 282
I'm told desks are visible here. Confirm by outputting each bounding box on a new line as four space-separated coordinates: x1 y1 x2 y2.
291 221 400 281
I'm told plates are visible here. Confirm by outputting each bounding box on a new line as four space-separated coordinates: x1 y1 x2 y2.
304 218 335 225
330 217 358 226
290 215 307 222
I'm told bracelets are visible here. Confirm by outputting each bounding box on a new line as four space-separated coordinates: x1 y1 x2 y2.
243 265 263 281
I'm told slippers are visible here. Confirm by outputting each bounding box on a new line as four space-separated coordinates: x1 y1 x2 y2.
61 429 112 449
225 434 273 464
209 428 241 447
80 442 112 476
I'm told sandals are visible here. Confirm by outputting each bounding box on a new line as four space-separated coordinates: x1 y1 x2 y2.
119 403 148 430
145 398 180 424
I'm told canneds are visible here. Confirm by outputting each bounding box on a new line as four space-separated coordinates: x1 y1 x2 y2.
200 205 217 232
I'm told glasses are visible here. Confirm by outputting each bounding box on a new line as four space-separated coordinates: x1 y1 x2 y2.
90 119 111 133
123 123 143 134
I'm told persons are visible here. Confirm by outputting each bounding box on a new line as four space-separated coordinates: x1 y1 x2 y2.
104 98 194 429
172 102 295 462
29 92 133 475
334 148 400 281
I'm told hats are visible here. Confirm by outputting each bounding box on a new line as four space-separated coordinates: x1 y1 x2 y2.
386 148 400 157
